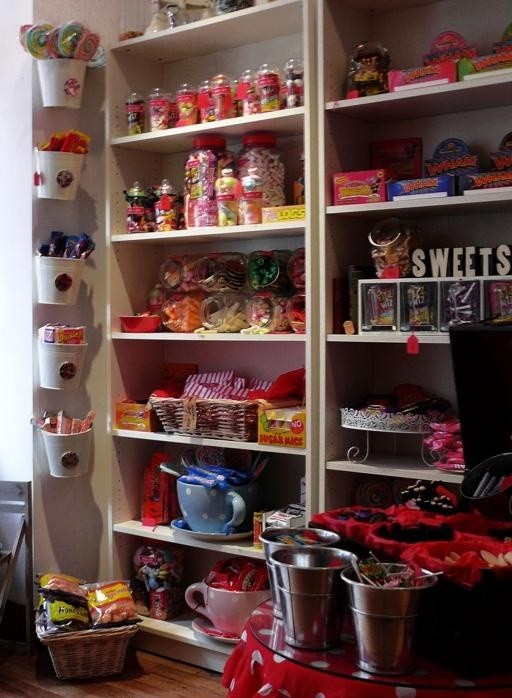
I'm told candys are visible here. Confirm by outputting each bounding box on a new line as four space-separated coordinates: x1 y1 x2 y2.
18 19 100 59
131 131 304 234
132 78 304 135
350 556 423 587
159 443 270 481
147 247 304 333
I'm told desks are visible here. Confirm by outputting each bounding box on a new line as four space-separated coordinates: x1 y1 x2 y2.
221 598 512 698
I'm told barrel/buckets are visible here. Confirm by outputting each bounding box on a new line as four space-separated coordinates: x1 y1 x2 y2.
269 613 287 652
265 545 358 653
339 561 438 679
40 428 94 480
258 529 340 616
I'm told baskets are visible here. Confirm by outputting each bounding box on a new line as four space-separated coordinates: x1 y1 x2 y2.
35 605 138 682
148 396 302 445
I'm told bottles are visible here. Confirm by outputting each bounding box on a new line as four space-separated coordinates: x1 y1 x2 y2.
160 246 306 335
215 170 238 227
127 181 148 234
239 132 286 224
292 152 305 205
364 217 423 279
147 282 162 317
242 165 262 223
183 134 238 229
154 177 180 232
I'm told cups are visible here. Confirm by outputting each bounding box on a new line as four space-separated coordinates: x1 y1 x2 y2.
126 92 146 135
184 576 275 636
175 479 260 534
169 58 304 129
263 525 440 681
41 423 96 478
146 87 169 132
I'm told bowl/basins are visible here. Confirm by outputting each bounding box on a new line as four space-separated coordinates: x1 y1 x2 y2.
119 316 162 333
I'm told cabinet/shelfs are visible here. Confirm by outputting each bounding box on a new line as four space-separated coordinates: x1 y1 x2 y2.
105 0 318 672
320 0 512 521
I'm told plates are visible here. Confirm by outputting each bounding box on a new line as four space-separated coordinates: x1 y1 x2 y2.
191 614 242 645
171 516 253 541
